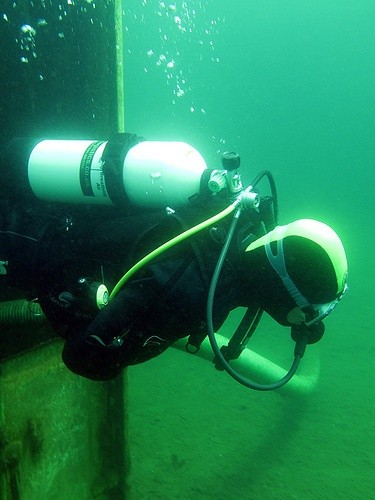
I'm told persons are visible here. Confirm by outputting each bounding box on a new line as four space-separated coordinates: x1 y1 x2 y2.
1 135 348 381
